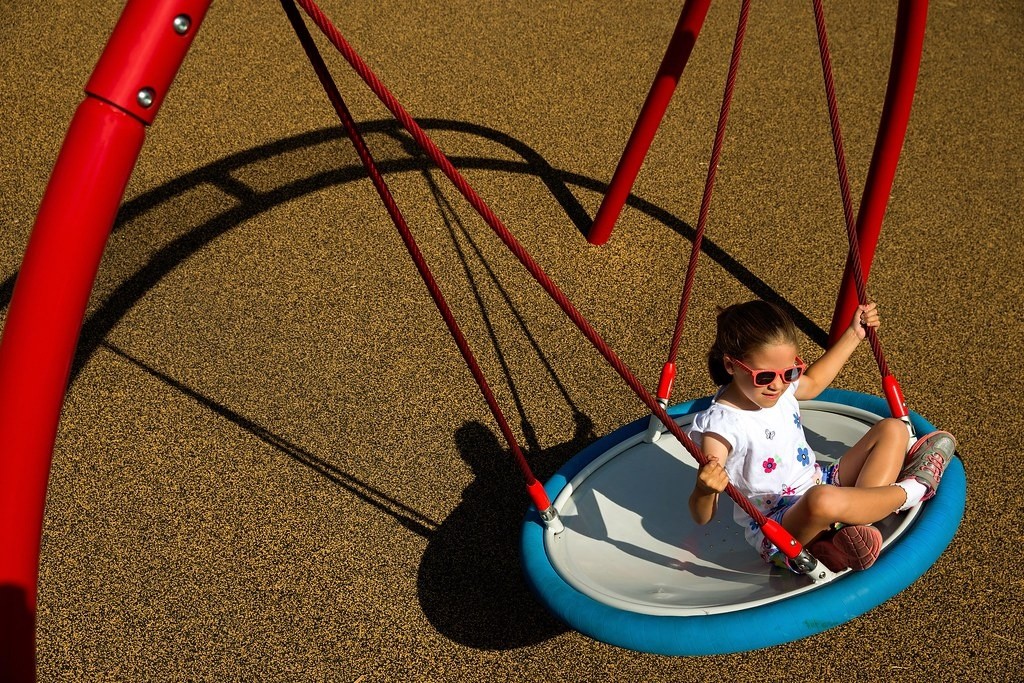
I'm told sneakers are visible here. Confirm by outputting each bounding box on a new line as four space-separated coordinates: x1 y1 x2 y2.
896 431 955 502
810 526 883 573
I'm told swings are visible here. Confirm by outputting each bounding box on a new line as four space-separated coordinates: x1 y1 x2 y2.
280 0 968 656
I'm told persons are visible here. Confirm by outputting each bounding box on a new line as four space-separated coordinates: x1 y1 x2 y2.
689 300 957 573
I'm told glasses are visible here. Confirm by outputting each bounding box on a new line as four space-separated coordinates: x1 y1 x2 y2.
724 353 806 386
